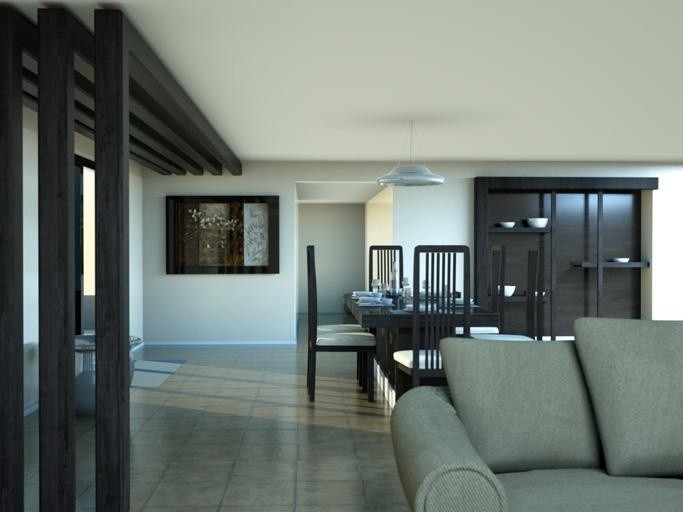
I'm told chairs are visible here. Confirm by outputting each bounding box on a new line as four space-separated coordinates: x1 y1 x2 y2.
470 251 538 340
456 250 500 333
393 245 471 399
307 244 377 402
369 245 402 293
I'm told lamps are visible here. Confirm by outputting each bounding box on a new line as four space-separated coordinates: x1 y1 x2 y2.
378 121 446 186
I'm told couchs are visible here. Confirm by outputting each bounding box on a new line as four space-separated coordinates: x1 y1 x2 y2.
388 318 683 512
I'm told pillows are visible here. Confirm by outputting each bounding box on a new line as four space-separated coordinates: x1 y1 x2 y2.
572 317 683 477
438 337 605 473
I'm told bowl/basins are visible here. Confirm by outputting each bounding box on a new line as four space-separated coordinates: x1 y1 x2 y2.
382 298 393 305
353 291 372 298
451 298 473 303
359 297 381 303
613 257 630 263
405 304 426 310
527 218 548 228
499 221 515 228
498 285 516 297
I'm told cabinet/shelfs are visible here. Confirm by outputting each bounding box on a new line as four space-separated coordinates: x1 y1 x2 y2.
474 175 658 340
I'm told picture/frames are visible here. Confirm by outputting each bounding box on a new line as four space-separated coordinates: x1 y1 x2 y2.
164 195 280 274
